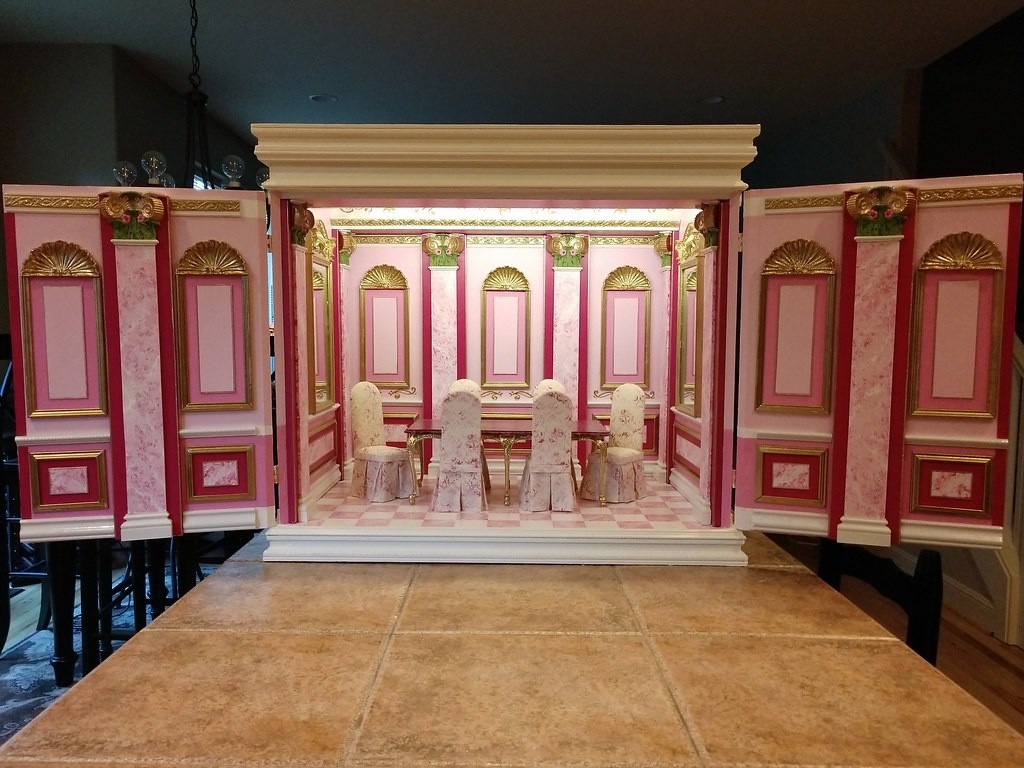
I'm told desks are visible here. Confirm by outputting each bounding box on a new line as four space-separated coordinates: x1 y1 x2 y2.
405 420 610 507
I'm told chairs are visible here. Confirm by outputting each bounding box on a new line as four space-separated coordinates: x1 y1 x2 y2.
348 381 420 504
449 379 481 402
531 379 566 398
518 390 578 512
581 383 646 504
431 390 490 512
